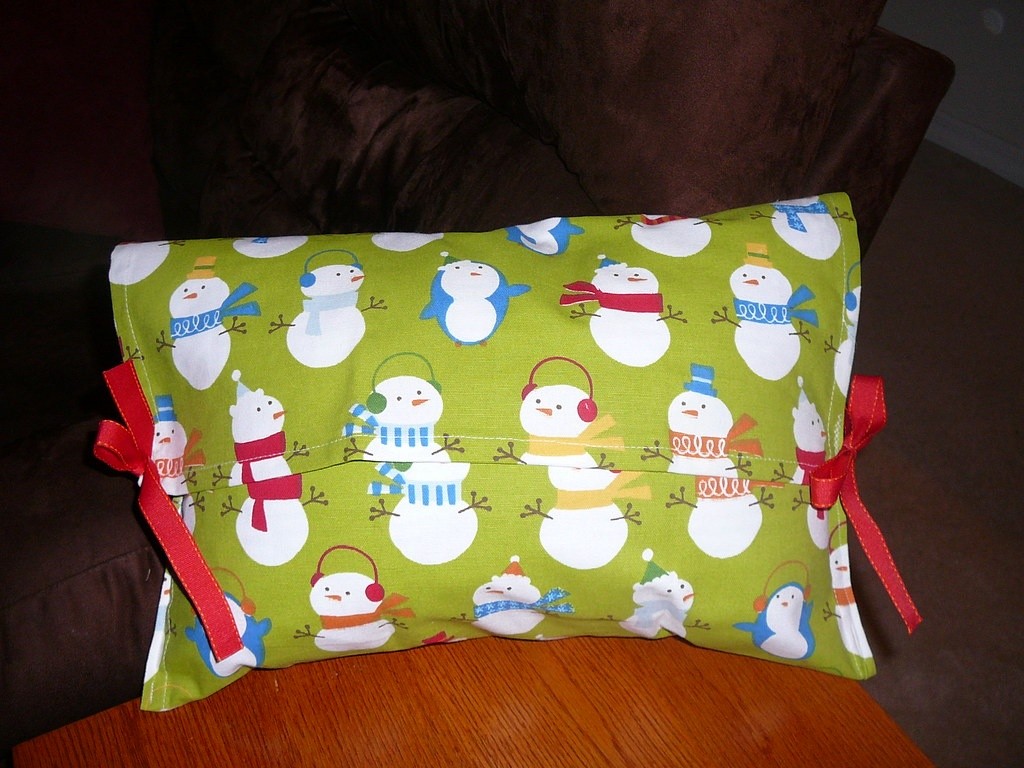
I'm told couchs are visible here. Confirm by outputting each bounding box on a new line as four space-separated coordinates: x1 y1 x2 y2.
0 0 956 747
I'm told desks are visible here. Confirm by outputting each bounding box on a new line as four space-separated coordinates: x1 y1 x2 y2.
16 635 929 766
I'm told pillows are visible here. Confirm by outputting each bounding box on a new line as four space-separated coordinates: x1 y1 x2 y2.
486 0 888 218
108 191 885 711
